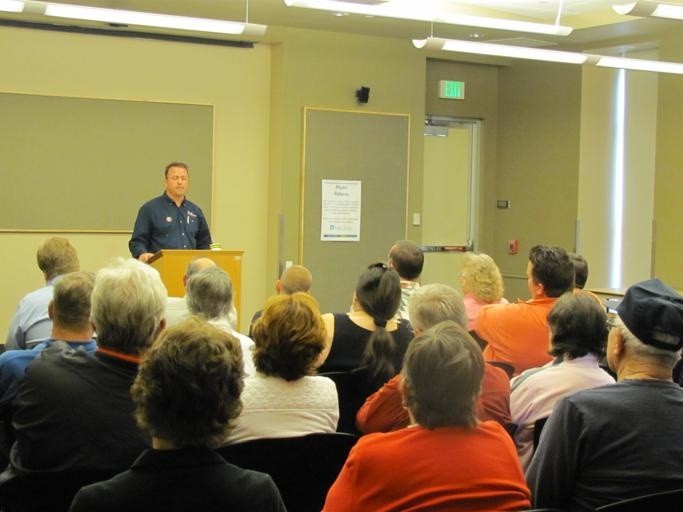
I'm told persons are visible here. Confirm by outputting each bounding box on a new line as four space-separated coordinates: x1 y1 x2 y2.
1 237 683 512
129 163 213 262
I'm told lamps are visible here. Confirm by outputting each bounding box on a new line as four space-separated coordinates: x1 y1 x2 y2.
1 1 269 42
287 0 575 36
410 20 683 74
613 0 683 27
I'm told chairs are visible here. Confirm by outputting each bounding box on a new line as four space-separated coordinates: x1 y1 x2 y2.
487 361 515 379
533 415 549 452
596 486 683 511
217 431 357 511
468 329 488 351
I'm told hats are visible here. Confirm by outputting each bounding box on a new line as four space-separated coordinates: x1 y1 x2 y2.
602 277 683 351
